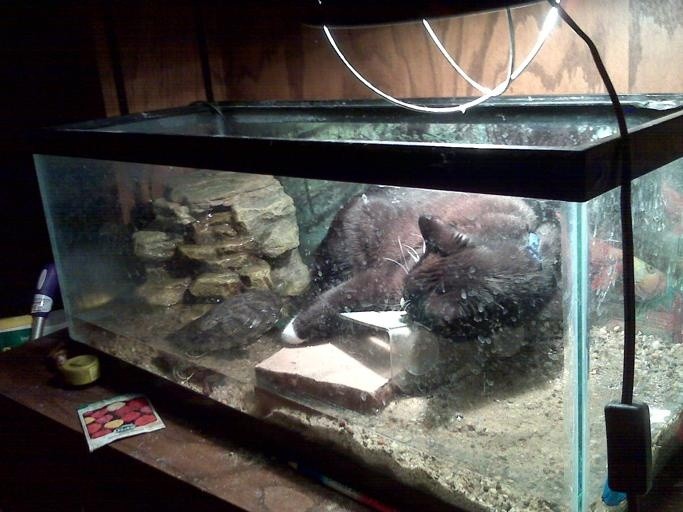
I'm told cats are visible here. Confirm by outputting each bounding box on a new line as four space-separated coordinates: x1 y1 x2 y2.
279 185 561 344
